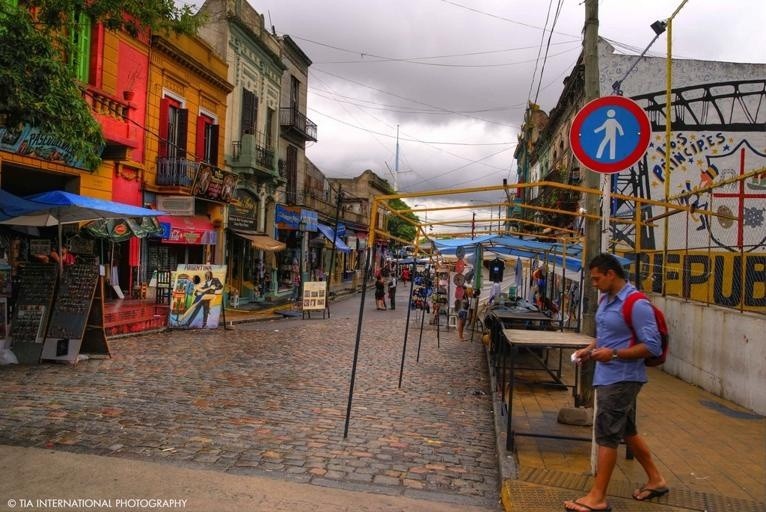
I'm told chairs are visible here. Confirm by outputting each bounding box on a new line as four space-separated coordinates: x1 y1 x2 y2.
155 268 172 306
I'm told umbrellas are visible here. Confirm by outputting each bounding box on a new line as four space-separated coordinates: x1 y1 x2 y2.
0 188 171 276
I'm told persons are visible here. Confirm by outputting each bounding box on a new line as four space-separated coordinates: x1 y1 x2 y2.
179 270 223 329
457 286 469 342
402 268 409 287
562 253 669 512
264 271 273 292
531 270 545 303
374 273 396 311
288 271 302 302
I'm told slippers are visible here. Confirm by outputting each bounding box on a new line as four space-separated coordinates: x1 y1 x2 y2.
632 483 670 501
564 496 612 512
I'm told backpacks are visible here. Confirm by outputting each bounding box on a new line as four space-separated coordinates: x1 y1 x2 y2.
599 292 669 367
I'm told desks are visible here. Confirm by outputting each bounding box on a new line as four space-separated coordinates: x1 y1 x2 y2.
489 308 636 460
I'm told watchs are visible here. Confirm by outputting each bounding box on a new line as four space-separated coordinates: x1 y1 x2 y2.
612 348 618 361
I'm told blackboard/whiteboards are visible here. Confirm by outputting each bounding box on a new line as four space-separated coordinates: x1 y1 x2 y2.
10 263 100 343
